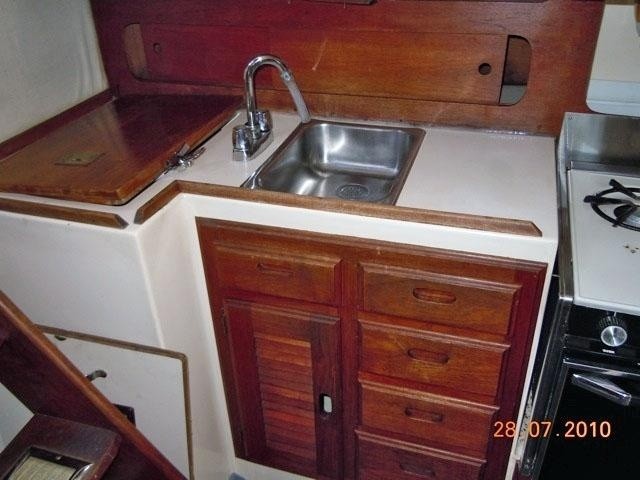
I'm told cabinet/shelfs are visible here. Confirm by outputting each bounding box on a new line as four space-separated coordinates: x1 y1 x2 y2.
182 194 553 480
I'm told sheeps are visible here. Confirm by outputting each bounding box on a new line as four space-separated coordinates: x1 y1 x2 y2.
231 52 312 162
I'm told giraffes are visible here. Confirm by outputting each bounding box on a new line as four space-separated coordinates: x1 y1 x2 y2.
566 168 640 318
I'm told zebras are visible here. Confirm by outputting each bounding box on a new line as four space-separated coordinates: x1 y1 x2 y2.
506 301 639 480
